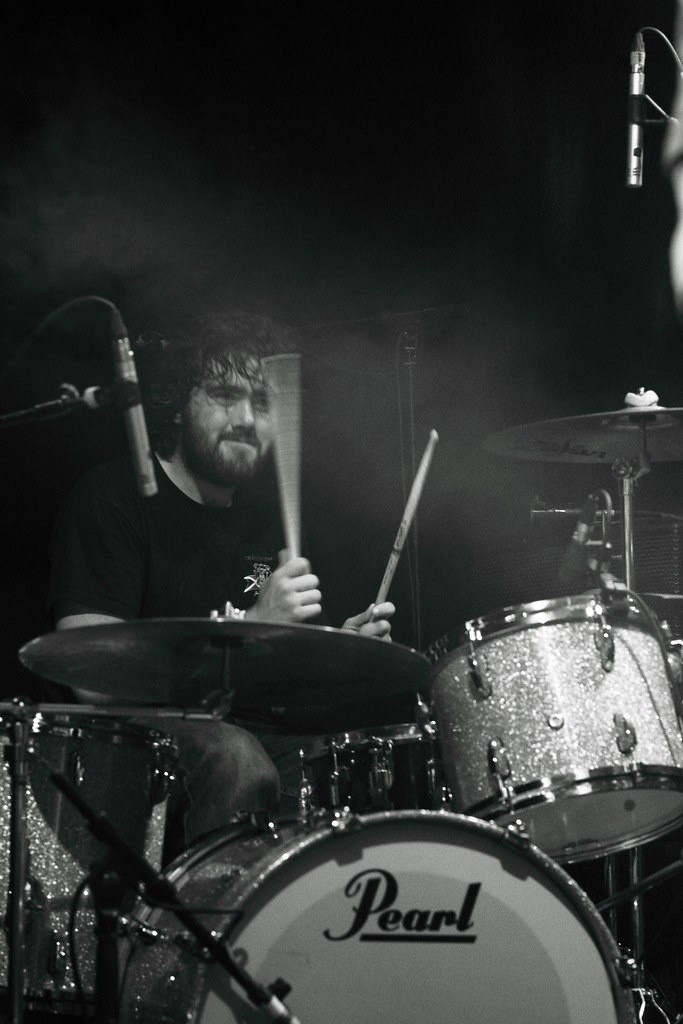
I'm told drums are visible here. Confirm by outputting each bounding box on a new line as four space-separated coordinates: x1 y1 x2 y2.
417 586 683 866
306 716 454 814
109 805 639 1024
0 714 172 1018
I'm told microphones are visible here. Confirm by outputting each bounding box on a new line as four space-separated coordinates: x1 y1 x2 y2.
112 309 158 497
559 493 599 585
626 33 645 188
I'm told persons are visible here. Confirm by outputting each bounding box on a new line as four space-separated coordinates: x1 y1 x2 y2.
48 309 440 848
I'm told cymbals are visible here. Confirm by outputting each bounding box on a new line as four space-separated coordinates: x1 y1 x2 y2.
19 617 433 731
480 405 683 464
